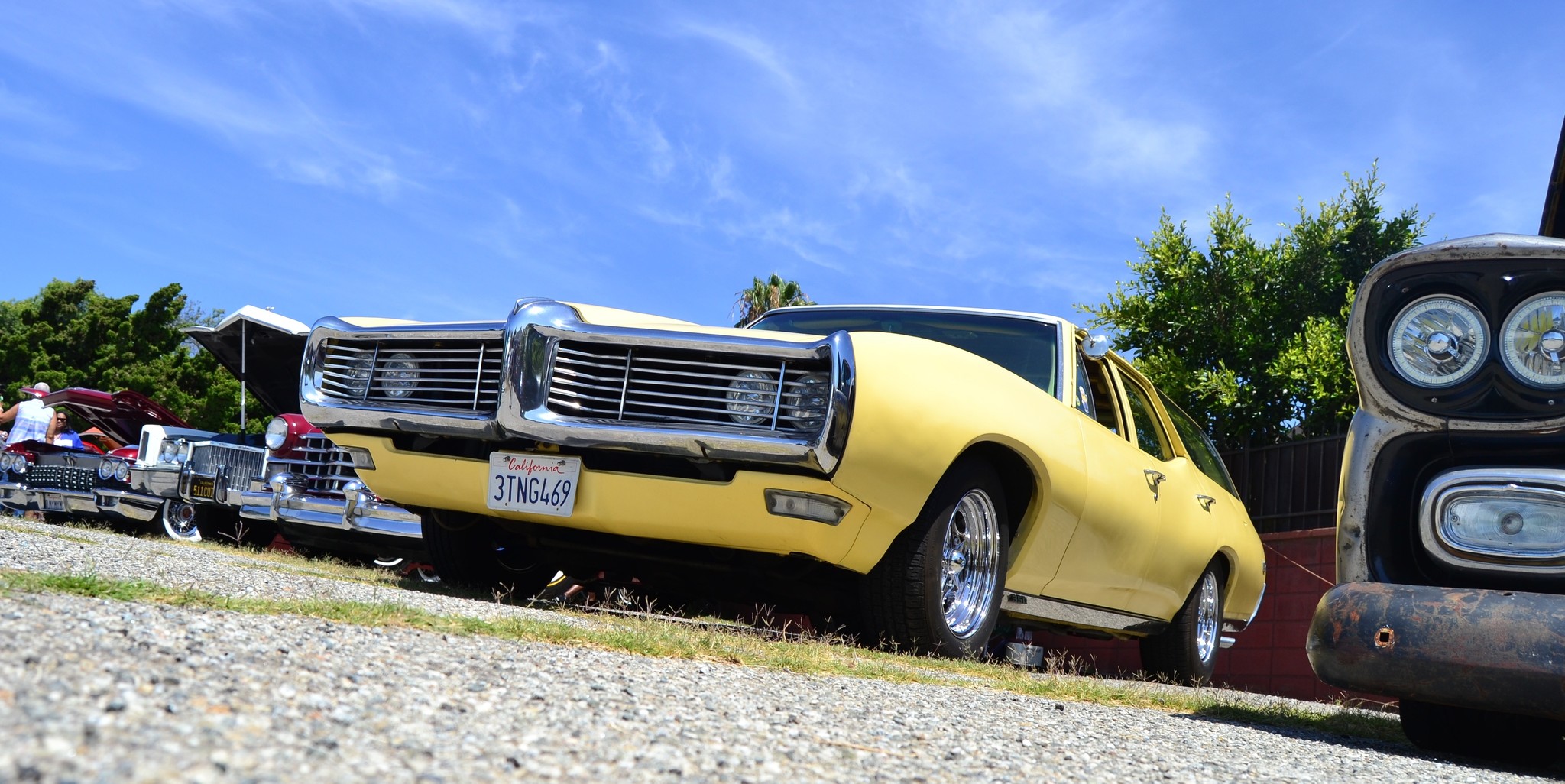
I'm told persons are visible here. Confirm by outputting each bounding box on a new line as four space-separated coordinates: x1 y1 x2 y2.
45 411 84 451
0 381 57 516
0 430 8 441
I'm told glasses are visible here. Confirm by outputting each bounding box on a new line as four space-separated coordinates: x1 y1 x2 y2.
57 417 67 422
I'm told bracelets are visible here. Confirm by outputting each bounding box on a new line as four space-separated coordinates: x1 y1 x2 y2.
0 407 4 412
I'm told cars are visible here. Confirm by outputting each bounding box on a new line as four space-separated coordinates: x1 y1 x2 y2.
299 297 1269 685
0 306 442 585
1304 117 1564 756
